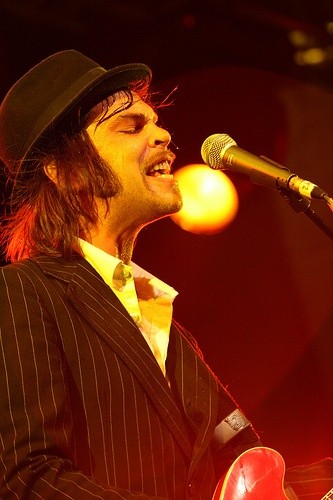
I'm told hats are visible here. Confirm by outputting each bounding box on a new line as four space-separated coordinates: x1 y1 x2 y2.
0 51 151 212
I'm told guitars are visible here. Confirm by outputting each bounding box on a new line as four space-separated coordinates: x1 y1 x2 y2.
211 446 333 500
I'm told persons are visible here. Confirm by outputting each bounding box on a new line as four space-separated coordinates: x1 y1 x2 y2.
0 51 300 500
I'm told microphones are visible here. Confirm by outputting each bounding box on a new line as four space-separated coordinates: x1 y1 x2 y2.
200 133 327 200
